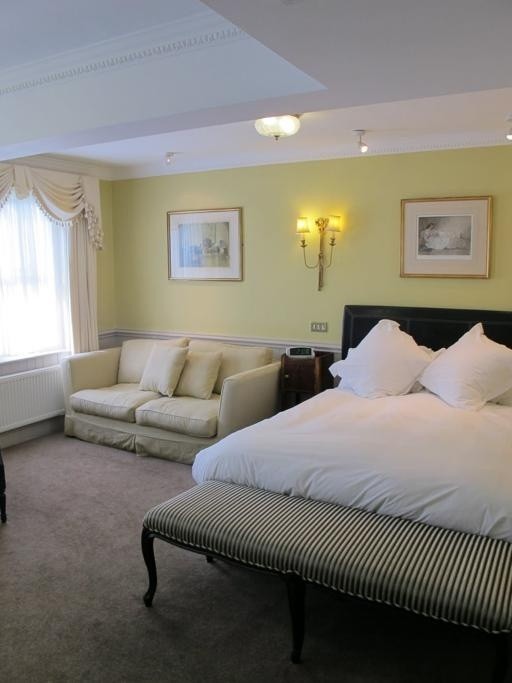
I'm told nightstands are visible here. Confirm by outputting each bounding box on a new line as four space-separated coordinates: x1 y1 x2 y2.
281 350 334 411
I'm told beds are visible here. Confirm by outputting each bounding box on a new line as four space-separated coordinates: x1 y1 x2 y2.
141 305 512 542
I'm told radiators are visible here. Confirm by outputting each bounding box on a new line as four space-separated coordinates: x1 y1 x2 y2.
0 365 65 434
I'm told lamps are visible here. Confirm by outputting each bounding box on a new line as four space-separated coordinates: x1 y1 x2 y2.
296 216 342 291
353 130 368 152
505 111 512 140
255 113 303 141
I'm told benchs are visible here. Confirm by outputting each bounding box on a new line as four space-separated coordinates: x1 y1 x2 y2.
141 480 512 683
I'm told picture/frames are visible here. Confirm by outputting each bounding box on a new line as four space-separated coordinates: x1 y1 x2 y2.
400 195 494 280
167 207 244 282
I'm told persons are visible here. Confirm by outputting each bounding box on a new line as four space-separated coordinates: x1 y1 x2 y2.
423 222 450 250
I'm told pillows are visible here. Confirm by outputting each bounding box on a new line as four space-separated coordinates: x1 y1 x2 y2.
117 337 273 399
328 319 512 411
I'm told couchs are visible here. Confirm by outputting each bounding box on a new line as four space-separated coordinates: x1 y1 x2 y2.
60 346 281 465
